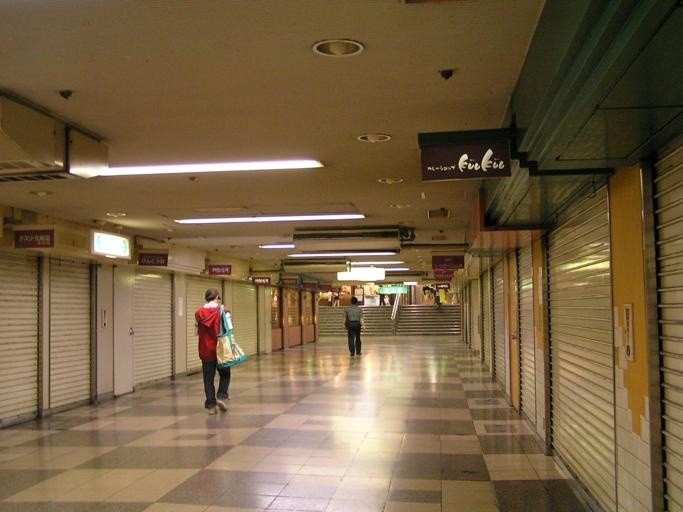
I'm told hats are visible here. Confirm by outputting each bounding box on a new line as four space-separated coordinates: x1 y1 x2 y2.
205 288 222 300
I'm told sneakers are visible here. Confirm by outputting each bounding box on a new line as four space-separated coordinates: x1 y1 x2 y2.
207 407 217 415
216 397 229 411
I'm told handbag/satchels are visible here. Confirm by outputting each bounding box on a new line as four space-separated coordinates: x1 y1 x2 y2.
344 318 352 329
216 329 248 372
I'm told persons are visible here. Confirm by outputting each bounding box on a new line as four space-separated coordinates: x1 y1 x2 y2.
332 292 339 306
379 294 384 306
193 288 235 416
343 297 365 356
434 289 440 305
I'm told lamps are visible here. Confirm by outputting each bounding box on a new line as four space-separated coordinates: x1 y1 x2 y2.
314 38 364 57
378 177 403 184
358 134 390 143
